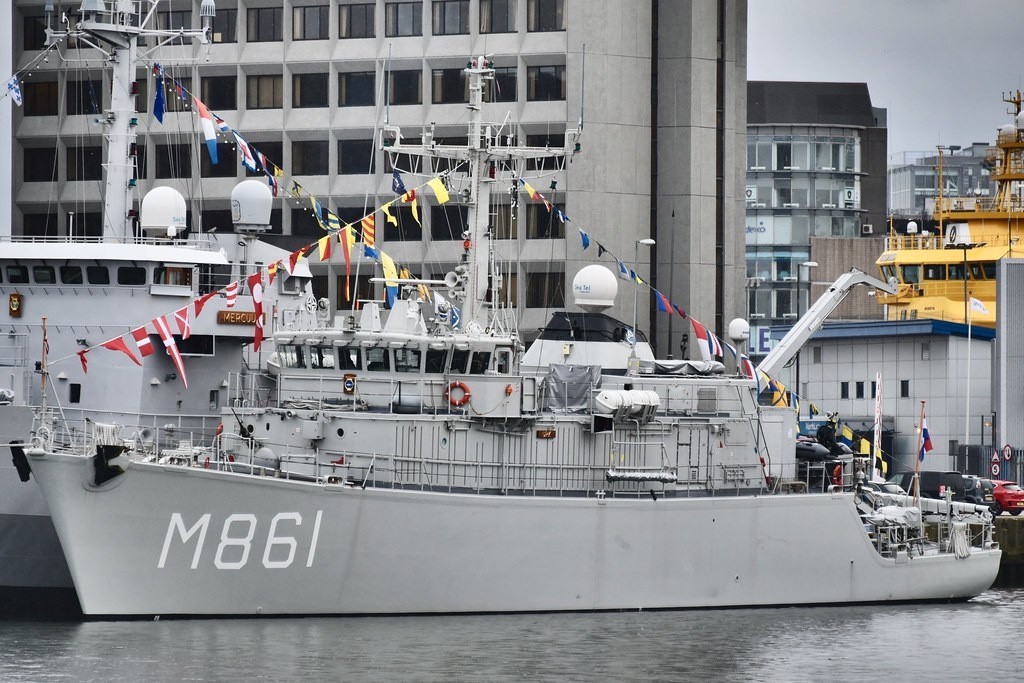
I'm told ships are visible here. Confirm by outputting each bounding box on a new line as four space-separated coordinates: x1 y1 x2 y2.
0 182 323 516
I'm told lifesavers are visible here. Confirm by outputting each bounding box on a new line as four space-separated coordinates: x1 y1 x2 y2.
445 382 471 406
833 465 843 485
216 423 223 436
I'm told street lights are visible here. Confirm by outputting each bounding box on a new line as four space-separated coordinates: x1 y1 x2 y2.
629 239 654 380
796 261 818 420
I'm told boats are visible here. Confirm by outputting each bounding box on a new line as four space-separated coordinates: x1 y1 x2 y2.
20 50 1002 616
875 90 1024 327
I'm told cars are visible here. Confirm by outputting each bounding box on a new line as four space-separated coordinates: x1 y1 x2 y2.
887 470 1024 517
